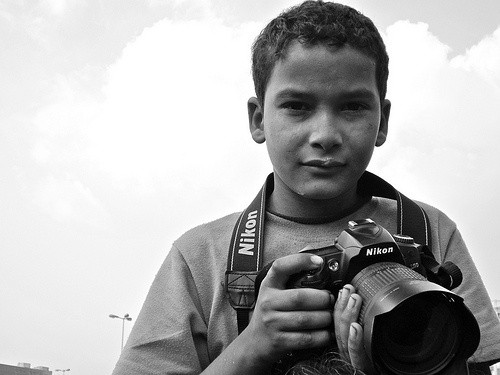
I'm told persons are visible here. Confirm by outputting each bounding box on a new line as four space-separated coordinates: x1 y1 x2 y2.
111 0 500 375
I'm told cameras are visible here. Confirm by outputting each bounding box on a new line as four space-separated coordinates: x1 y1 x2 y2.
285 217 480 375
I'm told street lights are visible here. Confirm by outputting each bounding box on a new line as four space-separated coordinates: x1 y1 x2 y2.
108 313 133 350
55 368 71 375
